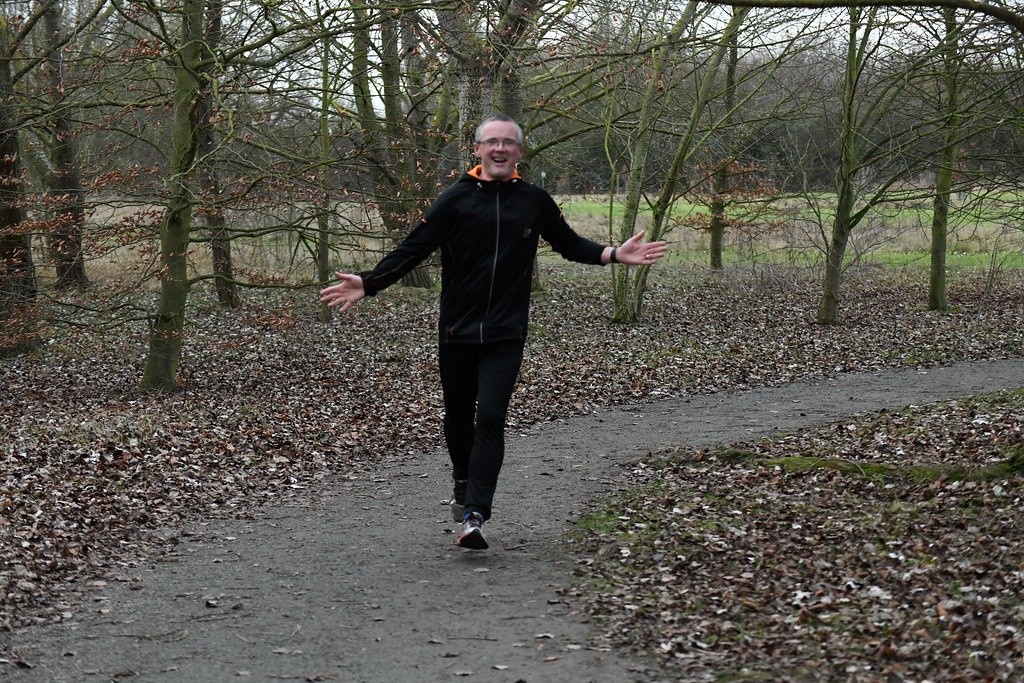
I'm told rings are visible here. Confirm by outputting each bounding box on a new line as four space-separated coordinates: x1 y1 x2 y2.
646 253 650 259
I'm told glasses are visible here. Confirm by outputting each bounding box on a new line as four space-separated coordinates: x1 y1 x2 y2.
477 138 521 149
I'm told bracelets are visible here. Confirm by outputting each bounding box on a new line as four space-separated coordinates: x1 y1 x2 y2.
610 246 620 264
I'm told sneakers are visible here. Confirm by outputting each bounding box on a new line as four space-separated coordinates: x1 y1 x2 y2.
449 486 465 522
454 510 489 550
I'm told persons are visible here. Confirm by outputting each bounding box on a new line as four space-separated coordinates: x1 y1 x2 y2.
318 113 667 549
